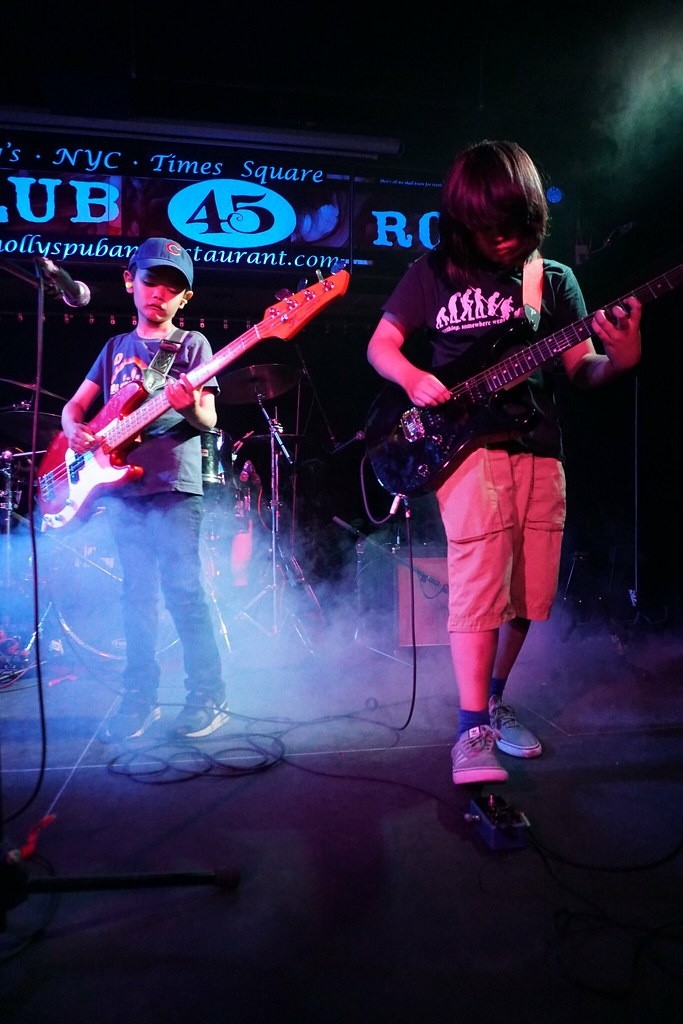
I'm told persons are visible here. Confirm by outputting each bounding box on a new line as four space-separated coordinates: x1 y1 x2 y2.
60 236 232 741
365 140 642 784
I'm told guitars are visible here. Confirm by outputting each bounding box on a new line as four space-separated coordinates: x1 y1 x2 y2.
366 267 683 495
32 271 348 535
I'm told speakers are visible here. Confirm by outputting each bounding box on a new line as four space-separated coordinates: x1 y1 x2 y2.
372 543 453 648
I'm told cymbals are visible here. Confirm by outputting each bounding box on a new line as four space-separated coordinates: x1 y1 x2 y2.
0 409 64 431
0 377 69 402
216 363 304 406
244 432 306 445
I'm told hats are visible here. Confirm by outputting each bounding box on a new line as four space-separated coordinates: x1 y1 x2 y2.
127 238 193 290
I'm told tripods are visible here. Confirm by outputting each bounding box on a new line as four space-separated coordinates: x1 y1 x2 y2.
220 393 440 672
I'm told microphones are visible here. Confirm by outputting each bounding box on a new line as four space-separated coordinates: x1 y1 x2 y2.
36 256 91 308
441 584 450 594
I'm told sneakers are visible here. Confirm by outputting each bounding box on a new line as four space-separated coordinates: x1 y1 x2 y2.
489 694 542 757
170 692 231 737
450 725 509 785
106 694 160 738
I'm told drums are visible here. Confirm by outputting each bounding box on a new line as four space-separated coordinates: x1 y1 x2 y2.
200 427 235 498
48 504 217 663
